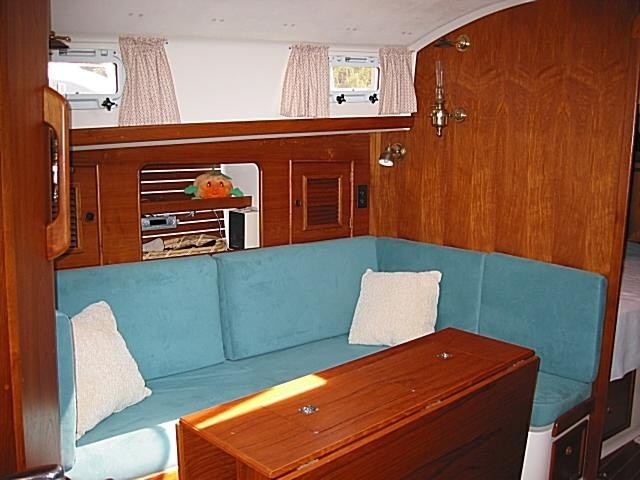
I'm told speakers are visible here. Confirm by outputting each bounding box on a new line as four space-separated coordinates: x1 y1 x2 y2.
229 208 260 250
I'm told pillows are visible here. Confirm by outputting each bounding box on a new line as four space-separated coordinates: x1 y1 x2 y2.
346 265 445 347
68 300 154 442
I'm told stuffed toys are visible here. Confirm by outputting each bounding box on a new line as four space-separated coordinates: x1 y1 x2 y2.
184 165 245 200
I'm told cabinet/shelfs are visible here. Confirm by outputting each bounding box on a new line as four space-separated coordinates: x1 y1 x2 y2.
287 159 355 245
549 397 594 480
50 167 101 269
601 370 636 440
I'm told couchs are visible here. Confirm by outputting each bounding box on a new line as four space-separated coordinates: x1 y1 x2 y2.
54 235 608 480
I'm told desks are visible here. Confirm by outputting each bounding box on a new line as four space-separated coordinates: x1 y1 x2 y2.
173 327 541 480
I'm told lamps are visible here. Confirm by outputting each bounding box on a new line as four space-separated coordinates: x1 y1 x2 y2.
427 33 470 138
378 141 407 168
